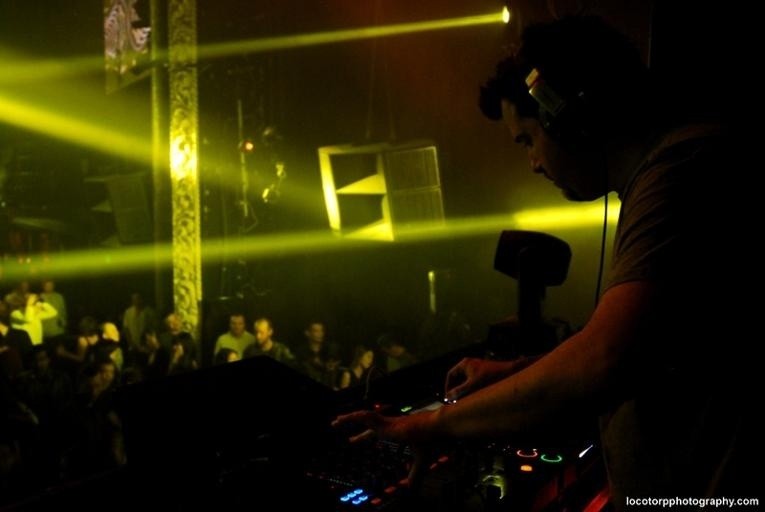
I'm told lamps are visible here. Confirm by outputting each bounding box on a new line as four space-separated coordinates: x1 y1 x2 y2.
483 229 573 347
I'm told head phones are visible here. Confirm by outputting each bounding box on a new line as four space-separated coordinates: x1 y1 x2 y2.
510 47 615 160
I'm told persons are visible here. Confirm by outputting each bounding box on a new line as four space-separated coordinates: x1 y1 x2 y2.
0 265 417 475
328 15 765 512
